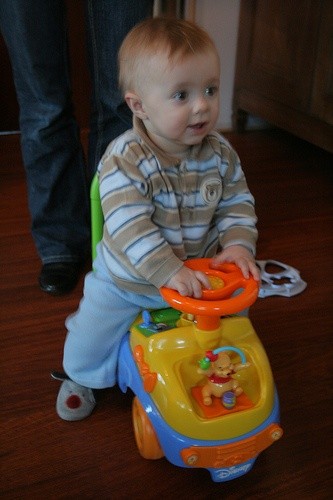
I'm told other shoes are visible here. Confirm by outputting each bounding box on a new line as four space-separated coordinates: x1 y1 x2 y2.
51 371 96 421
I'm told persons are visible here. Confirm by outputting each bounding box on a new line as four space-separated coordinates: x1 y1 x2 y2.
56 16 262 422
0 0 156 296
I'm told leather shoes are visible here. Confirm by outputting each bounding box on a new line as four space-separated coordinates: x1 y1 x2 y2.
38 259 88 295
